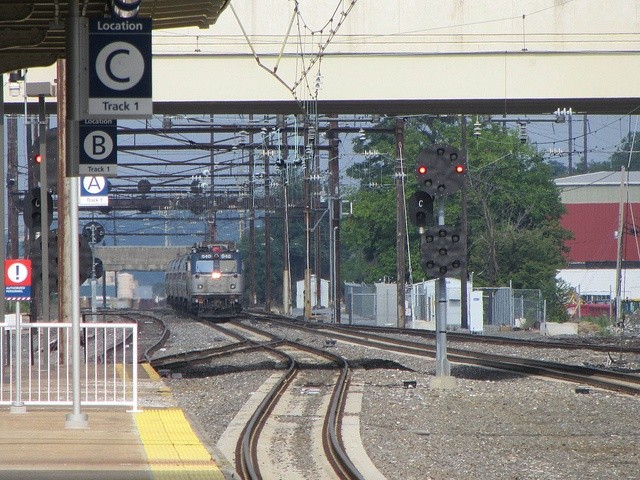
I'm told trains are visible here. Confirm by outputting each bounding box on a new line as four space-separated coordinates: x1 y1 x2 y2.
165 240 245 319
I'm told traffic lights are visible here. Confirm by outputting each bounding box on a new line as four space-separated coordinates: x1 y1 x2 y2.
32 144 41 178
